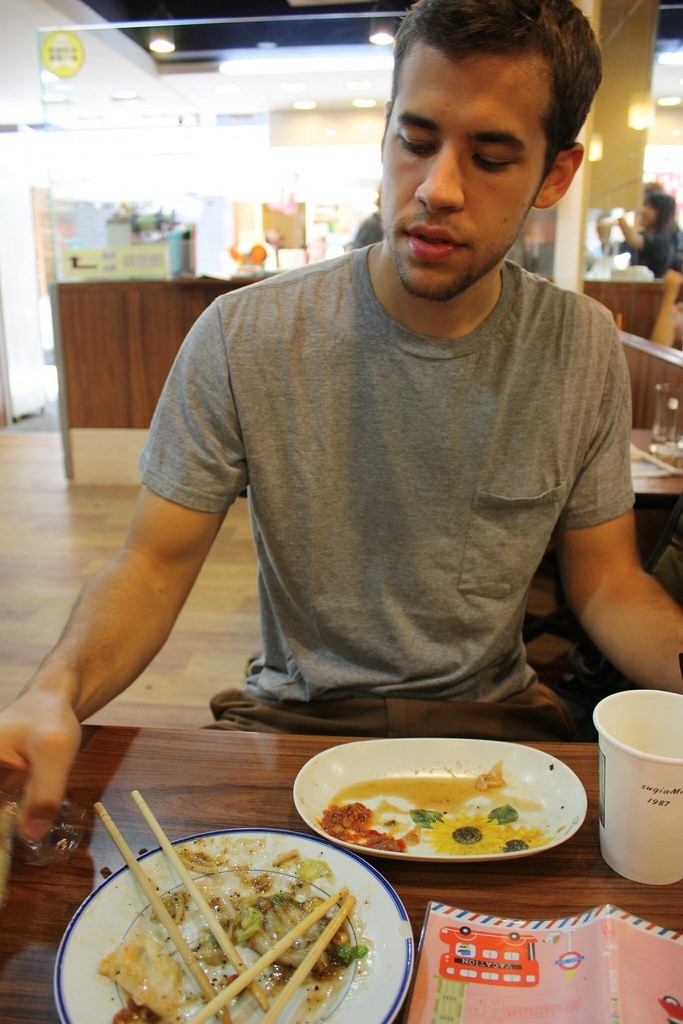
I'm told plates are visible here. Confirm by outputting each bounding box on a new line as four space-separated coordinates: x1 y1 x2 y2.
292 738 587 862
53 827 415 1024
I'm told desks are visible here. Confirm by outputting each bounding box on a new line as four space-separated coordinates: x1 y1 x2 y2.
0 715 683 1024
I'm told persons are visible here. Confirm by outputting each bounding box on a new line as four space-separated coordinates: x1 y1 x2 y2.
352 186 384 249
643 182 683 251
0 0 683 842
598 193 676 278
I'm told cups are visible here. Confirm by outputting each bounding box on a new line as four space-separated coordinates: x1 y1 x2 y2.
592 689 683 885
649 381 681 446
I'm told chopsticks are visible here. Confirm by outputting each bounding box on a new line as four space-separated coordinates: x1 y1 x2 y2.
187 887 355 1024
92 789 269 1024
